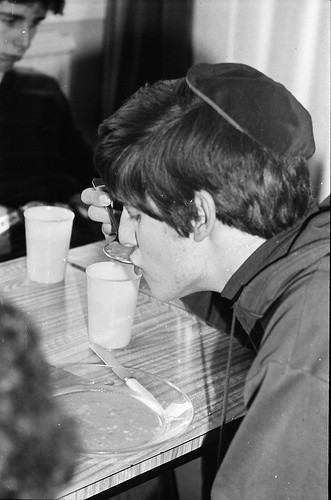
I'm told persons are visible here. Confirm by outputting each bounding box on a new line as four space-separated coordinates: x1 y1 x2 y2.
81 62 331 500
0 0 108 262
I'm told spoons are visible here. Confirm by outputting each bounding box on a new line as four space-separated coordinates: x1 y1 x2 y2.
91 178 134 264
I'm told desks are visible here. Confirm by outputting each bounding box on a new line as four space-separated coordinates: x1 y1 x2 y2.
0 241 254 500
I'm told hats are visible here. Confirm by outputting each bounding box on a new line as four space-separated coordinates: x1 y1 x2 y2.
187 64 315 158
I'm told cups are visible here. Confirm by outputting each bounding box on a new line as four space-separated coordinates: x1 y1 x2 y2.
86 261 145 349
24 206 75 285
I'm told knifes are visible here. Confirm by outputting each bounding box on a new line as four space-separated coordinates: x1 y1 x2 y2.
87 341 168 421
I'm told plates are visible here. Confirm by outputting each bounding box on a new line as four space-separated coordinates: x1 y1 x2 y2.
27 365 194 457
